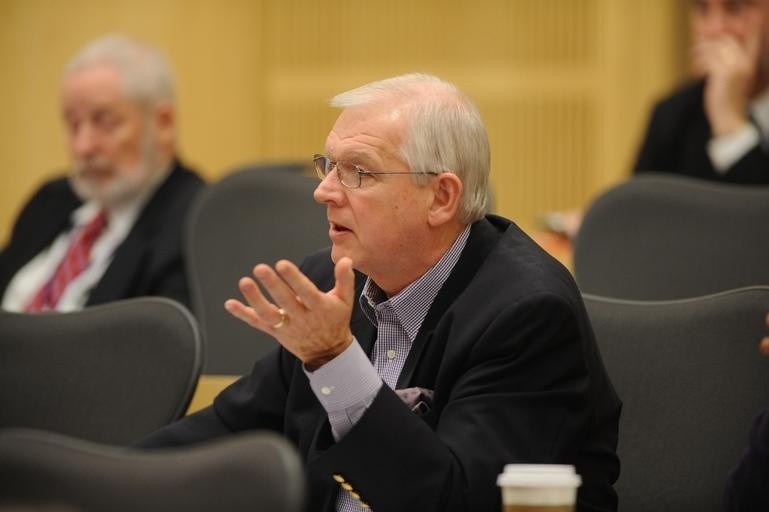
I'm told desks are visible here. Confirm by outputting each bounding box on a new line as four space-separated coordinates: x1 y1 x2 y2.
181 375 241 417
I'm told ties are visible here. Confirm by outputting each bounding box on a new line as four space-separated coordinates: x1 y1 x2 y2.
24 207 111 314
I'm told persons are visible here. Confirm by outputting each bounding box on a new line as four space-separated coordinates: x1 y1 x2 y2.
633 0 769 183
0 38 207 313
120 78 620 512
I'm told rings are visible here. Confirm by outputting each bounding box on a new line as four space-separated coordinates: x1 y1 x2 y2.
273 308 289 329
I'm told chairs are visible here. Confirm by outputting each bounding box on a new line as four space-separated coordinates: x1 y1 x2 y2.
580 285 769 510
0 427 307 511
0 295 204 449
179 162 334 376
572 174 767 304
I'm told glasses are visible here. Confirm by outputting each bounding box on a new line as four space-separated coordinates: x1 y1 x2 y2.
313 153 438 188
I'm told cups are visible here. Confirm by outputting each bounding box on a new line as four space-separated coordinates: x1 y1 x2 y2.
498 462 582 512
